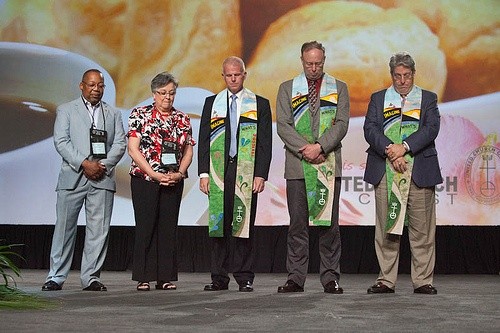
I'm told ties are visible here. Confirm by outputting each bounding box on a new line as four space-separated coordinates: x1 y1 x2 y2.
309 80 317 112
229 95 237 158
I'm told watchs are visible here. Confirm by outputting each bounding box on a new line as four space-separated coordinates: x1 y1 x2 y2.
402 143 408 152
177 171 185 181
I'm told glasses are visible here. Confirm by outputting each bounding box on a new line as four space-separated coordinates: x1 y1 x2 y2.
82 81 106 90
155 90 175 97
303 58 324 68
393 71 411 80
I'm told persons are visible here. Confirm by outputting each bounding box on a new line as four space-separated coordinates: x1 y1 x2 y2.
275 41 351 293
127 72 196 291
363 53 444 294
199 56 272 292
42 68 126 291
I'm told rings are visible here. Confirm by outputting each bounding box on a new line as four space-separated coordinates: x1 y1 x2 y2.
399 163 401 165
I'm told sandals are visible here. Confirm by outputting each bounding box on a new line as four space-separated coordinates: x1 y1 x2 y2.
155 281 175 289
137 281 149 290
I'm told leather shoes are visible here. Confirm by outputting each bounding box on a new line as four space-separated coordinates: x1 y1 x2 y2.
239 280 253 291
42 280 61 290
324 280 343 293
414 284 437 294
204 282 228 290
83 281 107 291
278 279 303 292
368 282 394 293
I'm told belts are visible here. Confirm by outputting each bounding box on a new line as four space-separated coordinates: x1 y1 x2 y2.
229 157 237 163
